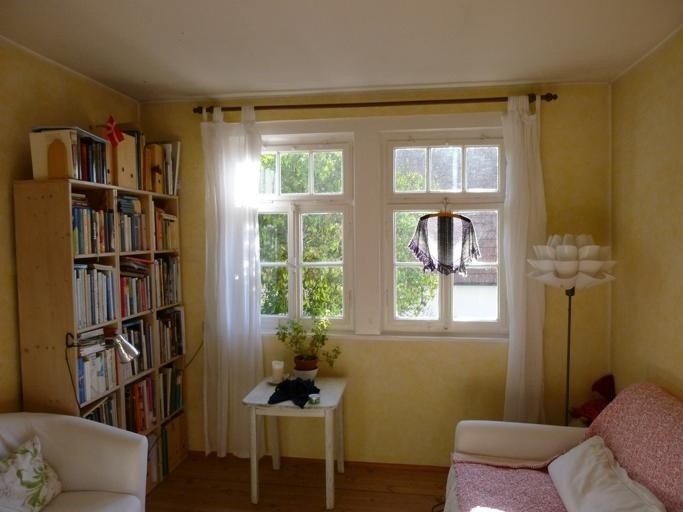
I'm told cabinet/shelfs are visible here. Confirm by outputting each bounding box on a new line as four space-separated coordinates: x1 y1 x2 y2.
12 174 191 491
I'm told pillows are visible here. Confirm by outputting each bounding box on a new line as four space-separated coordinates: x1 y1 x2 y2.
547 434 668 512
0 432 63 512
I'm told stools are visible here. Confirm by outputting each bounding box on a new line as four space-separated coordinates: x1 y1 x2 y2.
238 373 352 512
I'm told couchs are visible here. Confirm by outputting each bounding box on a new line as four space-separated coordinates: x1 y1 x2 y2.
439 381 681 512
0 411 150 512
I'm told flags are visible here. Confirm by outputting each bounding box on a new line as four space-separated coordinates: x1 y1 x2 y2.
102 115 125 148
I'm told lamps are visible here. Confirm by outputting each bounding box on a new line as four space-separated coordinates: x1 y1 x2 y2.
521 228 617 429
62 328 141 415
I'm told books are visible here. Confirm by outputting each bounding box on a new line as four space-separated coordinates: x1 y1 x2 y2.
70 194 114 327
117 196 180 316
77 309 186 433
143 413 186 491
31 129 181 195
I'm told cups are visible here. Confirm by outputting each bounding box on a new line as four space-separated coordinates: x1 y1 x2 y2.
271 360 283 384
308 393 320 404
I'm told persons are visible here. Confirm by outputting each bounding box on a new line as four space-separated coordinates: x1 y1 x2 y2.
568 374 616 429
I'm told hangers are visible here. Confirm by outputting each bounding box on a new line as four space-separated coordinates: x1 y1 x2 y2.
419 197 472 223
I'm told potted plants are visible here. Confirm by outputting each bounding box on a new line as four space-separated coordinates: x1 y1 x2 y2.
275 311 342 387
